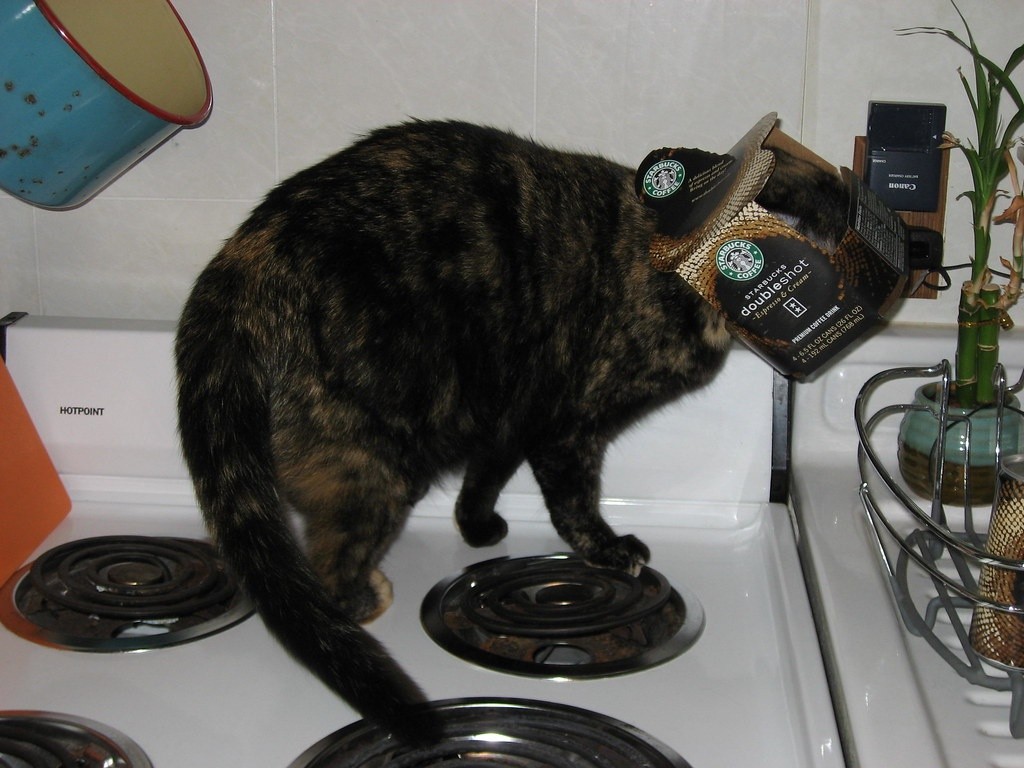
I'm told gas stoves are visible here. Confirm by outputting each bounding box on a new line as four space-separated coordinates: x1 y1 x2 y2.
0 312 843 768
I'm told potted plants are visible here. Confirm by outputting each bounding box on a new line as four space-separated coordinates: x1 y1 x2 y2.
898 0 1024 508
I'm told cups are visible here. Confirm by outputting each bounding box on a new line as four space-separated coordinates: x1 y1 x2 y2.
0 0 214 212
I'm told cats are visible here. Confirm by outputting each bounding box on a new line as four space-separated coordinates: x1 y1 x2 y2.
171 115 857 750
